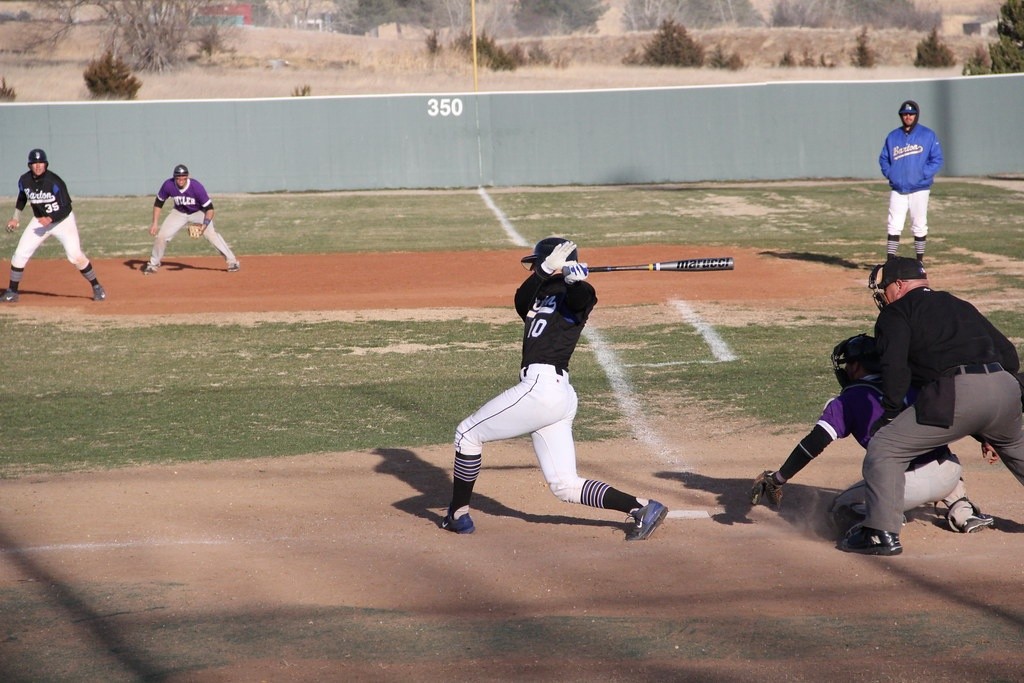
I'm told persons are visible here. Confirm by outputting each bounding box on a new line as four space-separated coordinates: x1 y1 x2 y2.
439 237 669 539
0 148 106 302
879 100 944 261
750 257 1024 555
142 164 240 275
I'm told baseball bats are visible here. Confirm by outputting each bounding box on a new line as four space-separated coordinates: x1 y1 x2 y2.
562 257 735 277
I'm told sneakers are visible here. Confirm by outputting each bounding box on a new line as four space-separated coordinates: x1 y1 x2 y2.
439 514 476 534
0 289 20 302
228 263 239 272
841 527 903 555
624 500 669 541
145 266 157 274
962 514 994 534
93 285 105 300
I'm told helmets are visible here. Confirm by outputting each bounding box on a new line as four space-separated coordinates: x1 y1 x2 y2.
845 335 883 372
173 165 189 176
28 149 47 167
521 236 577 271
898 103 918 113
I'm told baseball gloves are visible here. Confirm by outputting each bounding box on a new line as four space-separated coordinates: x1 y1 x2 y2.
752 470 783 510
188 224 204 238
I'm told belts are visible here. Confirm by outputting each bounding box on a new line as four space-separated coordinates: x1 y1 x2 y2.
519 365 570 381
953 363 1003 376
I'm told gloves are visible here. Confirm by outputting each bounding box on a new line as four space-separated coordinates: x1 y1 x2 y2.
564 262 588 284
540 241 577 275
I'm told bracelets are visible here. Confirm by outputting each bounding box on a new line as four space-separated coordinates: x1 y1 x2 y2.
203 219 211 225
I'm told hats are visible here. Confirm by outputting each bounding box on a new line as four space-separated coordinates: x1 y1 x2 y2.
877 256 927 290
